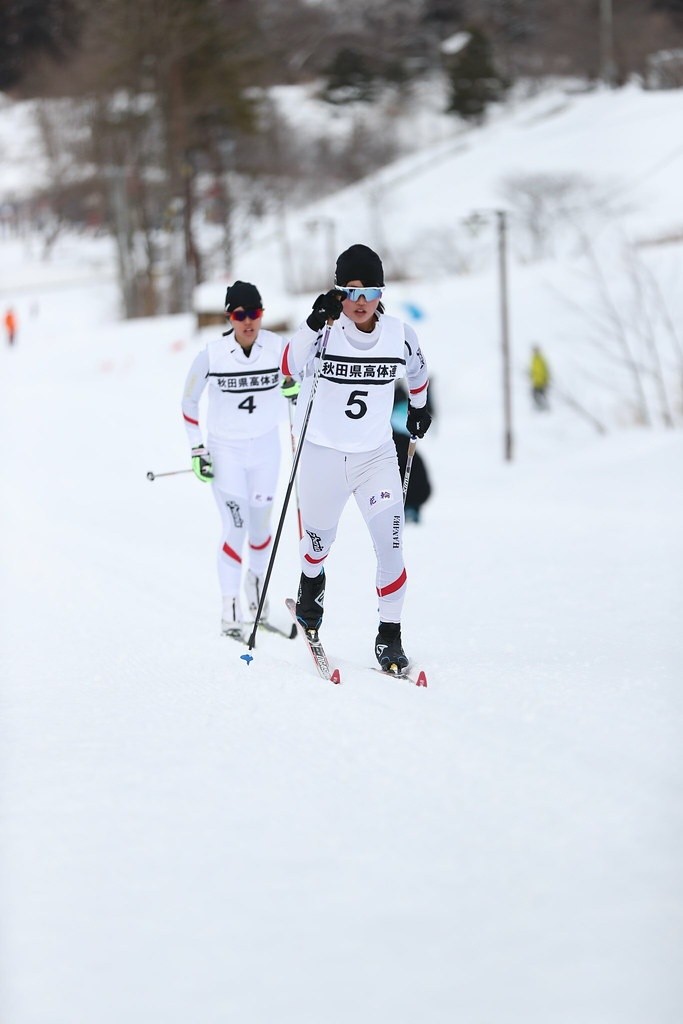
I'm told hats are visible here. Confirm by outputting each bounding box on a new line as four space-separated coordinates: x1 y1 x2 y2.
225 280 261 311
334 244 384 287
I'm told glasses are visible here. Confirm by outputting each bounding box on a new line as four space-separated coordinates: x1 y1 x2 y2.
226 308 264 321
335 285 386 302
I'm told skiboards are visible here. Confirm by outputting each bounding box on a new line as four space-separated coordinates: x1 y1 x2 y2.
229 598 430 689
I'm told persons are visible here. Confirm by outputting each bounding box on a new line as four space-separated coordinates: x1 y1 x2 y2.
180 281 305 634
311 7 514 119
5 309 17 346
531 347 549 412
282 244 432 674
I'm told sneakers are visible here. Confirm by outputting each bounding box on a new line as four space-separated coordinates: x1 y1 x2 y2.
375 621 410 676
296 566 326 629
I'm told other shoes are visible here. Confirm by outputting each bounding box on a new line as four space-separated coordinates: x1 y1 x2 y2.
221 620 244 637
243 569 267 618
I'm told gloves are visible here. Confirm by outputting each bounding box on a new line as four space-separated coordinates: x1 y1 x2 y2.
282 377 298 400
306 290 347 332
191 445 214 483
406 398 432 438
